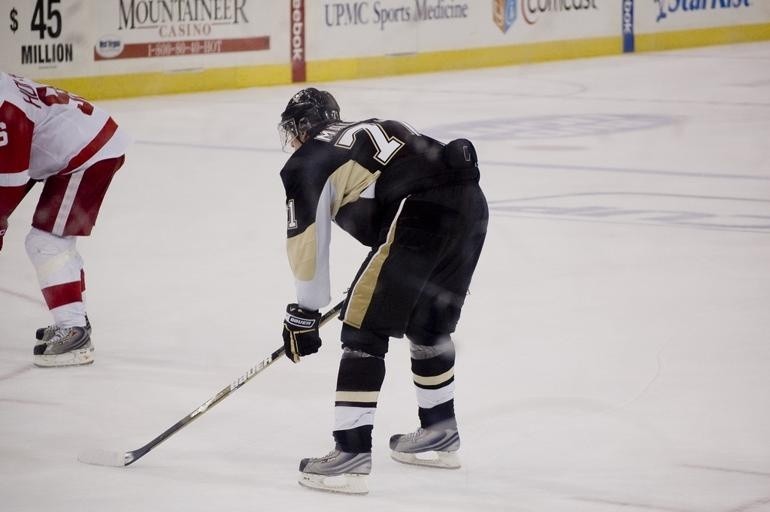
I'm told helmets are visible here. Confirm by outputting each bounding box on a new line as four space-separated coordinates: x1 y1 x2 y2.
278 87 339 155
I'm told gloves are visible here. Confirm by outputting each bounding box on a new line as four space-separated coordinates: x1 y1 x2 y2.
282 303 323 364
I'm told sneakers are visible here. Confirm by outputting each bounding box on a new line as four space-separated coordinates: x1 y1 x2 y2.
33 313 91 355
389 427 461 454
299 450 373 476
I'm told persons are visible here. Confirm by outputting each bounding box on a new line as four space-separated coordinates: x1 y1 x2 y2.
276 89 490 475
0 71 125 356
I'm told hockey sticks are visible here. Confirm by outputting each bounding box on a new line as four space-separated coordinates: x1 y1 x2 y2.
78 298 347 468
